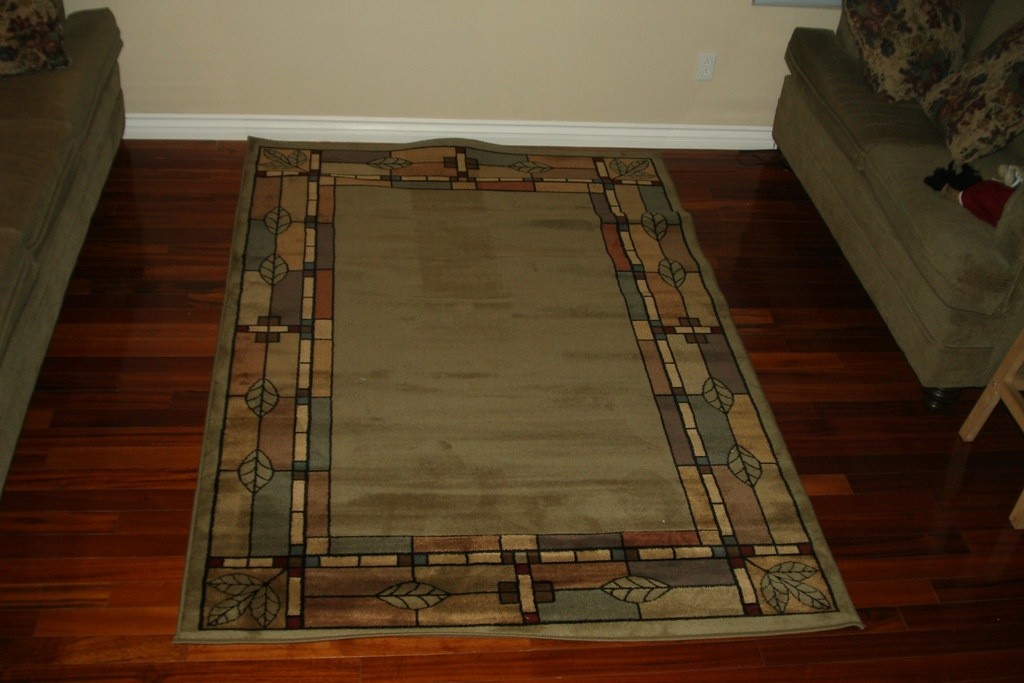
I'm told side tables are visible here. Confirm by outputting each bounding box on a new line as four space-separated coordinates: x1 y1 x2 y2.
958 329 1024 528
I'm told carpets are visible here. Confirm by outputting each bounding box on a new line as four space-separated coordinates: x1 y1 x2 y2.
171 135 862 645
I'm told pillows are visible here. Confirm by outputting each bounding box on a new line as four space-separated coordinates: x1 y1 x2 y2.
843 0 964 102
0 0 62 75
920 21 1024 167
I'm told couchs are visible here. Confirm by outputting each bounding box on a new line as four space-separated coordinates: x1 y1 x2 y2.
771 0 1024 389
0 0 125 489
